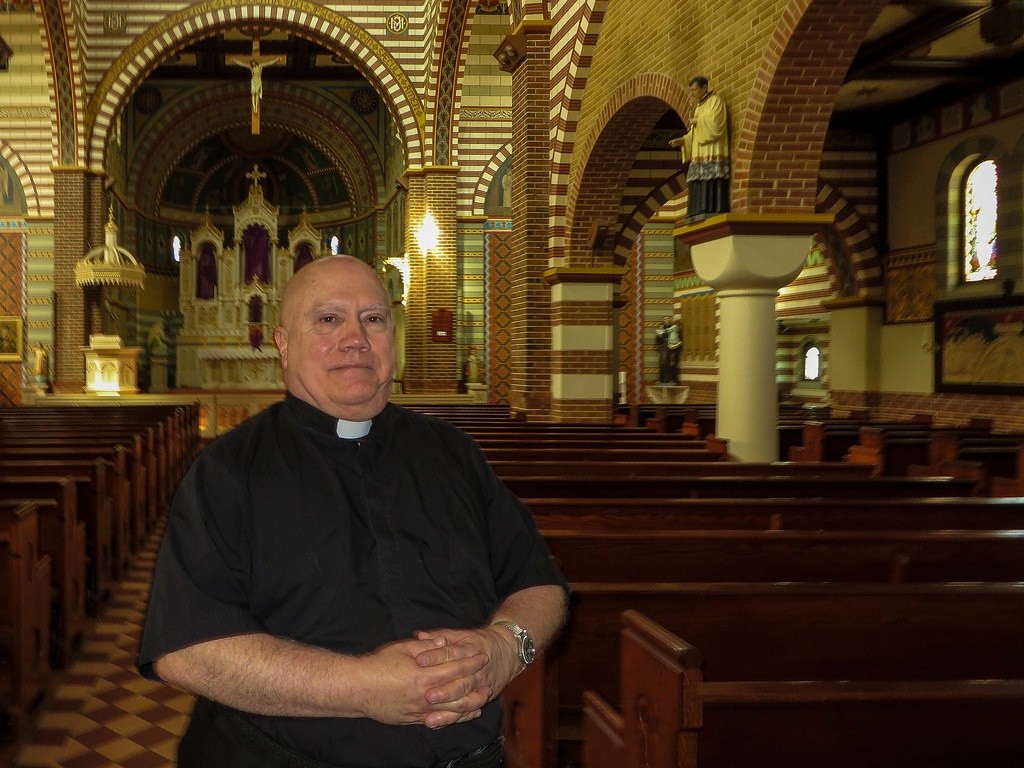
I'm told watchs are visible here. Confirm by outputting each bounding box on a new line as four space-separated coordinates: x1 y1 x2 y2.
495 620 536 676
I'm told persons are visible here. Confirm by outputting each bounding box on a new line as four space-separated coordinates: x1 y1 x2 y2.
28 341 48 383
465 348 478 382
655 314 682 385
668 76 731 218
147 320 169 355
233 58 279 113
136 254 570 768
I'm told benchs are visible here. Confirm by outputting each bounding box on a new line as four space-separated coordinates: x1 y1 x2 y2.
391 399 1024 768
0 403 197 765
